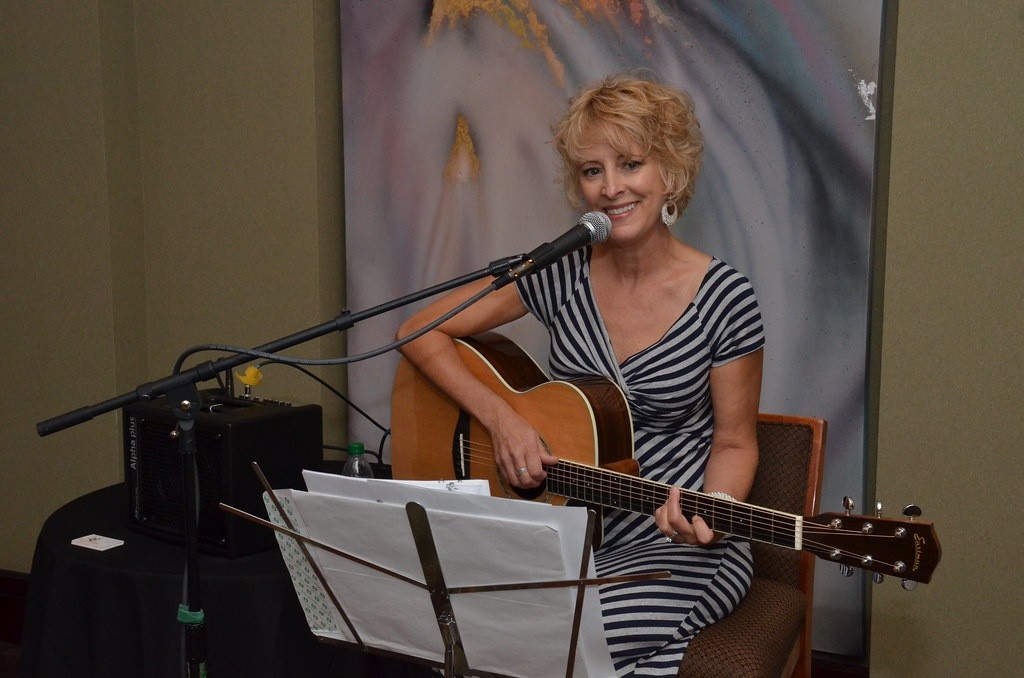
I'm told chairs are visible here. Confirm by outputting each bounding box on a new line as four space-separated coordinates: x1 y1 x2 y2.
677 412 829 678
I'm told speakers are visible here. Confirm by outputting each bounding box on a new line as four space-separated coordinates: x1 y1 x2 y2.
122 388 323 560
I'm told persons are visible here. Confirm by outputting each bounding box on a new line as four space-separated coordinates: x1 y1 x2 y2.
394 75 766 678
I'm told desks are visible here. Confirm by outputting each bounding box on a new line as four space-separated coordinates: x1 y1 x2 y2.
18 458 392 678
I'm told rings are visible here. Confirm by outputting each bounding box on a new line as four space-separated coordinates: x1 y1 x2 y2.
515 467 528 477
669 530 678 539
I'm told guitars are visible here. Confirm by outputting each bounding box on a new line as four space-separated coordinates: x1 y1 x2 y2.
388 332 943 592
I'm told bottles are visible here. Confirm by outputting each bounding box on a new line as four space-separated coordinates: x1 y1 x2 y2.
343 442 375 481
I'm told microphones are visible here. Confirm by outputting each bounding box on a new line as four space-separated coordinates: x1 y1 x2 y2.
490 211 612 290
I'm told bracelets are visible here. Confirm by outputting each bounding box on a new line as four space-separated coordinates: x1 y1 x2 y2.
709 492 736 502
